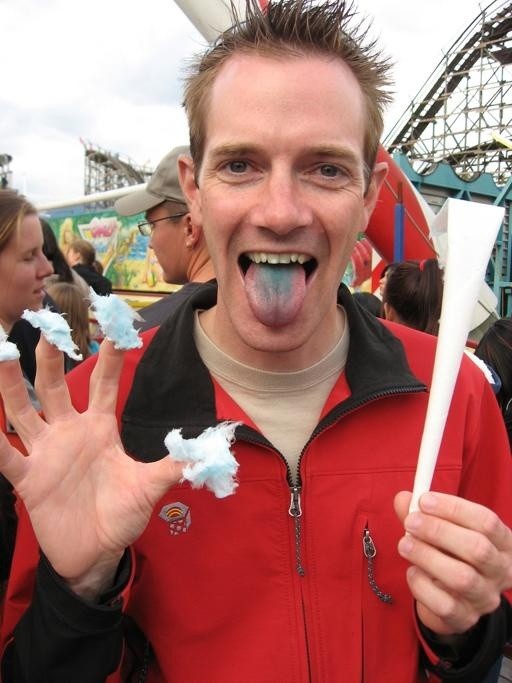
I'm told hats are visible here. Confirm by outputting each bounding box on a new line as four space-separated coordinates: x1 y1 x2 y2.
112 144 195 218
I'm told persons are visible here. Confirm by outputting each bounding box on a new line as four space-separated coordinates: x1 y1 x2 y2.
353 258 512 445
2 144 216 414
0 0 512 683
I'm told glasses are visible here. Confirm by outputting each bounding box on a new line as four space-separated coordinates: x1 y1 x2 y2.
135 211 188 236
